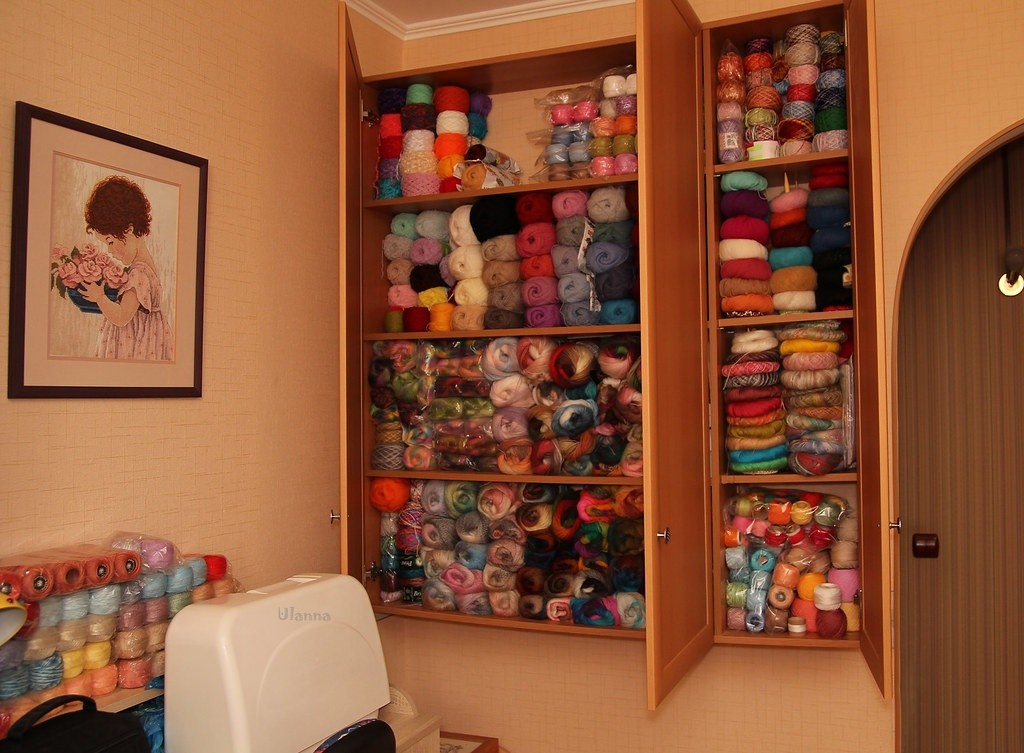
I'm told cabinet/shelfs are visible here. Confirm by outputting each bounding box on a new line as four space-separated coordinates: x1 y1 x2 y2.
331 0 890 712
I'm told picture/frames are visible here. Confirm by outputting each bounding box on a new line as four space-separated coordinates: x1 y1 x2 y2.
440 730 499 753
6 101 208 399
